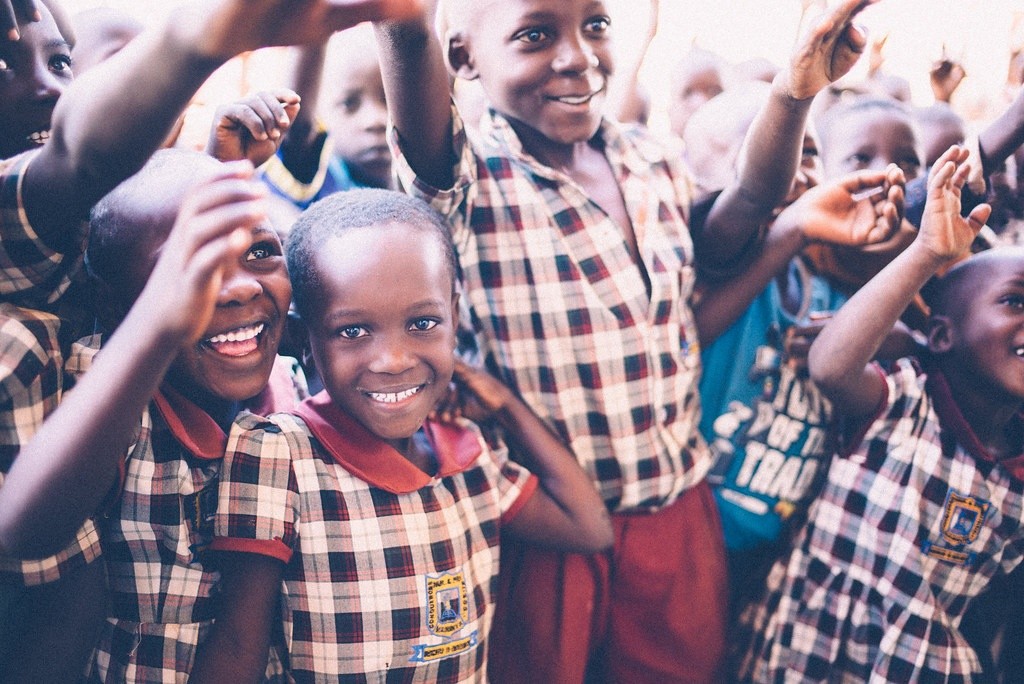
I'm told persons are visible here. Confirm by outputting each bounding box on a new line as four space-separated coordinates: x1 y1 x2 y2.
0 0 420 684
0 148 311 684
67 7 301 168
818 94 924 198
184 185 614 684
262 41 391 213
915 100 976 168
682 1 882 285
722 144 1024 684
616 1 1024 135
369 0 725 684
